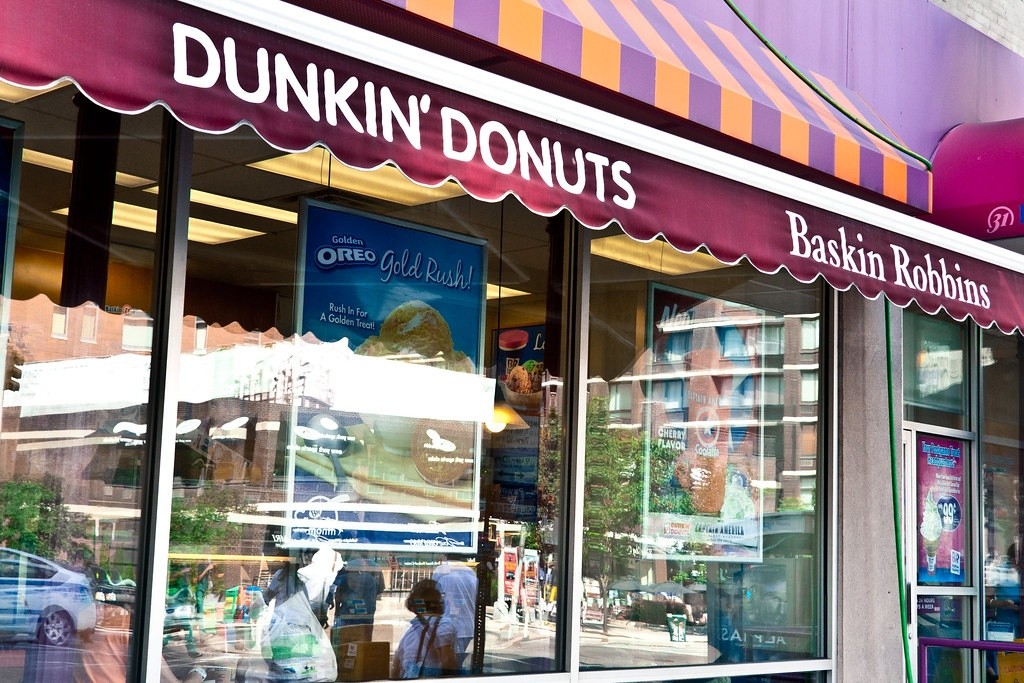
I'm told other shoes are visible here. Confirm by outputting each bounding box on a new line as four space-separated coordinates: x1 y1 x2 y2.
187 648 203 658
199 630 213 644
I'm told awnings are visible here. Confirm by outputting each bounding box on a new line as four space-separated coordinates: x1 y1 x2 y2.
931 117 1024 240
0 0 1023 333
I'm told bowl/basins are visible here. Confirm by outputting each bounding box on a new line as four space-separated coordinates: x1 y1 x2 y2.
374 414 417 456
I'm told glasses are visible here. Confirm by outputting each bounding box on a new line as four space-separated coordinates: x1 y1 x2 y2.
440 591 447 598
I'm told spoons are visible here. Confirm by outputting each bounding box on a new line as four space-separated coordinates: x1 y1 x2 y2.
304 414 362 502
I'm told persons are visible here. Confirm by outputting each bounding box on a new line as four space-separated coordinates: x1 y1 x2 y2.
194 562 224 641
985 543 1020 625
431 554 478 653
75 578 230 683
248 552 385 663
683 570 699 625
163 564 203 657
391 580 460 677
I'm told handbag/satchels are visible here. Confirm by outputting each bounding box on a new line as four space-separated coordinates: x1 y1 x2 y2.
244 592 338 683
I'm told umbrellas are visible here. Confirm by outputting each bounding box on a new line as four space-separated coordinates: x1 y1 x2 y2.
607 579 706 608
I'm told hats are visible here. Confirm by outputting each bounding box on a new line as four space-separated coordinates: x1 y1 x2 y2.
347 555 366 567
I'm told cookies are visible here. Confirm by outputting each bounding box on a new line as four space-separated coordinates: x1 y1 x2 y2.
411 416 471 486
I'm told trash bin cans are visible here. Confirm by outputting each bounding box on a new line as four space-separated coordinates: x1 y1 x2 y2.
665 613 688 643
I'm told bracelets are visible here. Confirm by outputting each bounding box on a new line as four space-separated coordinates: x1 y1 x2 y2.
188 668 207 680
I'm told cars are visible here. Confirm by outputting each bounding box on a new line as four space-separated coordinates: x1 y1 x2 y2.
0 546 98 647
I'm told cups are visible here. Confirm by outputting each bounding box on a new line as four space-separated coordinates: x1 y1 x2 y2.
680 350 736 519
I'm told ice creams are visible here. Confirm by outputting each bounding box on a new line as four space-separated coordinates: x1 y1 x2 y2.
920 490 943 575
